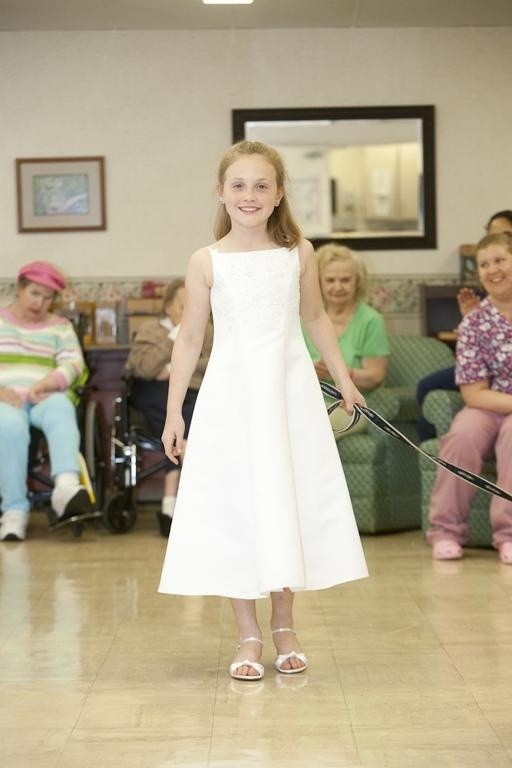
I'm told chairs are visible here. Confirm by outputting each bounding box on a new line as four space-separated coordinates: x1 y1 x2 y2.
415 365 498 549
340 334 458 536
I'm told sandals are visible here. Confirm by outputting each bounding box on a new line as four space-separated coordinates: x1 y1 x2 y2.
229 637 265 680
272 628 307 673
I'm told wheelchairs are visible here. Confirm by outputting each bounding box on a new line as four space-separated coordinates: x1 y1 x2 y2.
104 332 181 532
27 313 104 539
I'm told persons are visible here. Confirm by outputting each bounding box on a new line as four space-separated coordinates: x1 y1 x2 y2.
303 243 391 443
122 280 214 540
416 210 511 441
155 141 368 680
0 261 89 542
424 231 512 564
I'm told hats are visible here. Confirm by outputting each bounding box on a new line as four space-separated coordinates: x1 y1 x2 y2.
20 261 64 292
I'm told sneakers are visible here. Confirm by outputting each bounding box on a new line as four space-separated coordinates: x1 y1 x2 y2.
50 486 89 522
0 508 27 540
432 541 464 559
499 543 512 564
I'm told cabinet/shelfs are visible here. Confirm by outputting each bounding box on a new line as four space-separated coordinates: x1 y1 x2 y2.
29 348 171 506
418 283 458 347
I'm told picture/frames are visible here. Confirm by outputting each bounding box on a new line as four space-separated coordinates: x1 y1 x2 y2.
229 103 439 253
15 153 109 234
